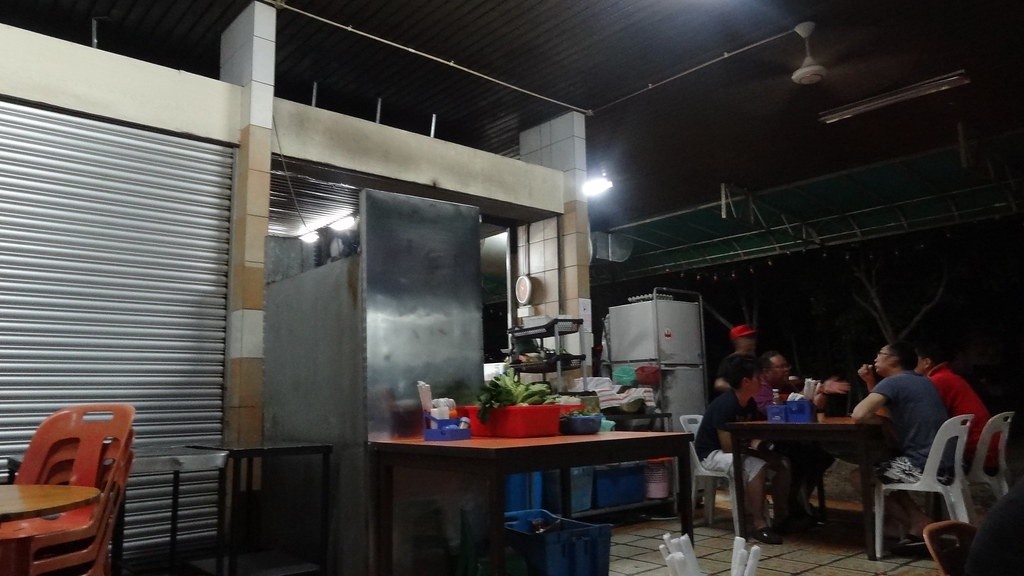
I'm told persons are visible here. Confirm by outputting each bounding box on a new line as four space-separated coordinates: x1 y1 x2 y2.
964 486 1024 576
695 324 851 544
851 341 1000 554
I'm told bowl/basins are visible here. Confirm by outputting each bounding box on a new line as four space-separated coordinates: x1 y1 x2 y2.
600 421 615 431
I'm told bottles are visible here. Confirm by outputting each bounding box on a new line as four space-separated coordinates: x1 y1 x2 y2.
458 417 470 430
772 388 781 405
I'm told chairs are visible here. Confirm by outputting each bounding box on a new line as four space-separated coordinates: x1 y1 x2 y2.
0 401 137 576
679 415 768 540
874 414 974 558
969 411 1015 499
923 520 977 576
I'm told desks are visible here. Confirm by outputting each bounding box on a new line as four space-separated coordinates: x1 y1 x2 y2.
366 433 696 576
610 413 674 432
170 439 334 576
0 484 101 526
719 418 882 562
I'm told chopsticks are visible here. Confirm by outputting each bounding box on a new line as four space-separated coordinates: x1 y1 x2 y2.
417 379 432 413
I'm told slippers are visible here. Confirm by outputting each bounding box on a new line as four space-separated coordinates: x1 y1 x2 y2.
891 534 926 555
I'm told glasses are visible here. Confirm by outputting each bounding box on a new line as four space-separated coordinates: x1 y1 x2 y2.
876 351 896 359
767 363 791 371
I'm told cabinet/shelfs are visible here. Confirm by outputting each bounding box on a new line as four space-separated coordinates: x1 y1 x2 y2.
508 315 588 392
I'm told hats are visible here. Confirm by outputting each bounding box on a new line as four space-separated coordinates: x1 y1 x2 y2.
730 324 758 341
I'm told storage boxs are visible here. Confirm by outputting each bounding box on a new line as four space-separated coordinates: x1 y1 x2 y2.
504 470 544 522
468 404 562 438
506 509 612 576
594 461 645 509
558 465 593 513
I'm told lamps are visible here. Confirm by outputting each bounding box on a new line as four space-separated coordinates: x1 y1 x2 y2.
816 69 973 125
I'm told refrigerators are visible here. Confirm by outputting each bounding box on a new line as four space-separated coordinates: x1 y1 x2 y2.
608 300 704 491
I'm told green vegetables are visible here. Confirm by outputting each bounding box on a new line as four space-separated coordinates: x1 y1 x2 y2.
560 408 595 419
475 372 560 425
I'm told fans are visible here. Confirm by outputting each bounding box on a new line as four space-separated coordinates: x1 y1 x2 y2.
727 21 907 115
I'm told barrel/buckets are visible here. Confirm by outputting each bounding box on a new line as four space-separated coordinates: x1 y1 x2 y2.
559 416 601 434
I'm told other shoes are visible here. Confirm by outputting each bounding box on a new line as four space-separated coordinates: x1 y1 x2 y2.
753 527 783 545
773 517 807 535
